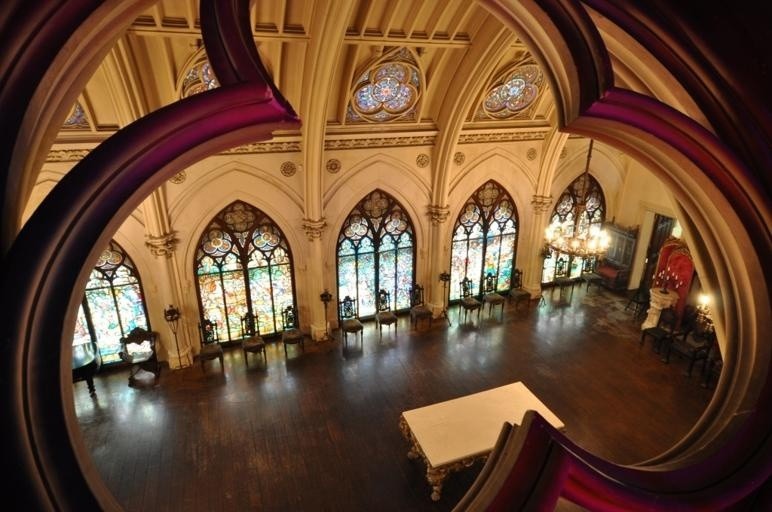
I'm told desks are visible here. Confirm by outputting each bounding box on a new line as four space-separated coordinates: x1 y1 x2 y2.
400 380 565 501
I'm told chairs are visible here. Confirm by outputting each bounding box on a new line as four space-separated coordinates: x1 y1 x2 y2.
551 258 603 295
120 327 162 388
638 308 717 380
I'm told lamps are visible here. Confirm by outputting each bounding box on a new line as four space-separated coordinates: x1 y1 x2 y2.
541 132 609 260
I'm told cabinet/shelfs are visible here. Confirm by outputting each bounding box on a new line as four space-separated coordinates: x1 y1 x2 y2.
592 222 639 292
